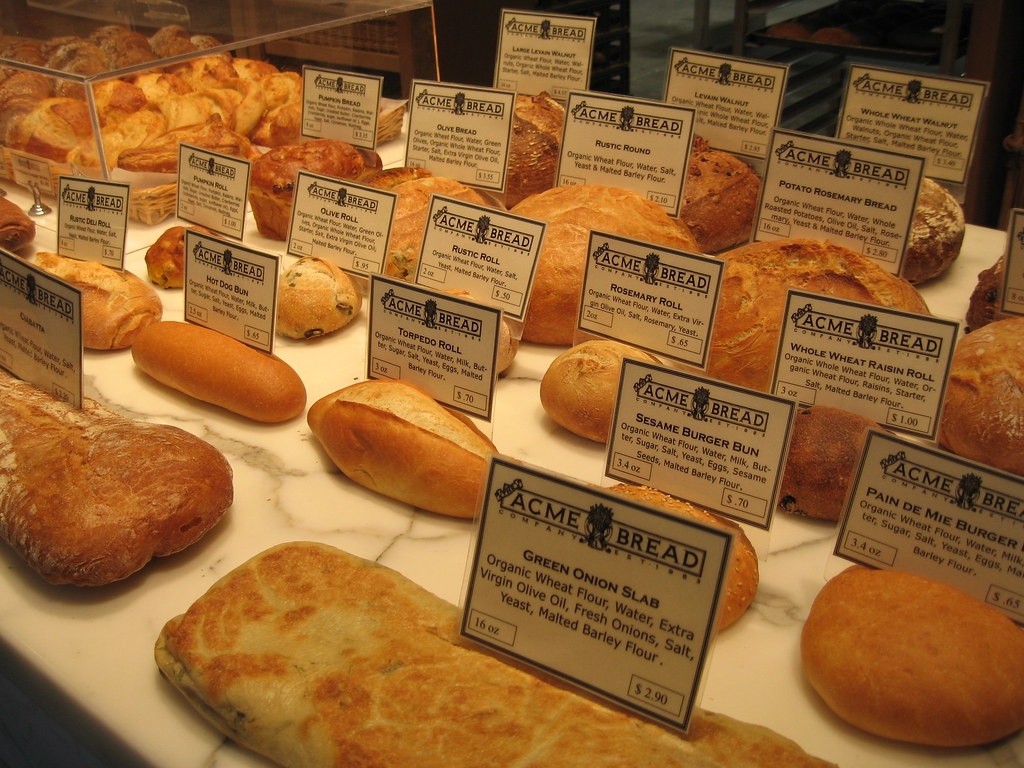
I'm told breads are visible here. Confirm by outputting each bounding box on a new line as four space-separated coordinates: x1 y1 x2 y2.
0 197 1024 768
139 90 1024 520
0 22 305 177
